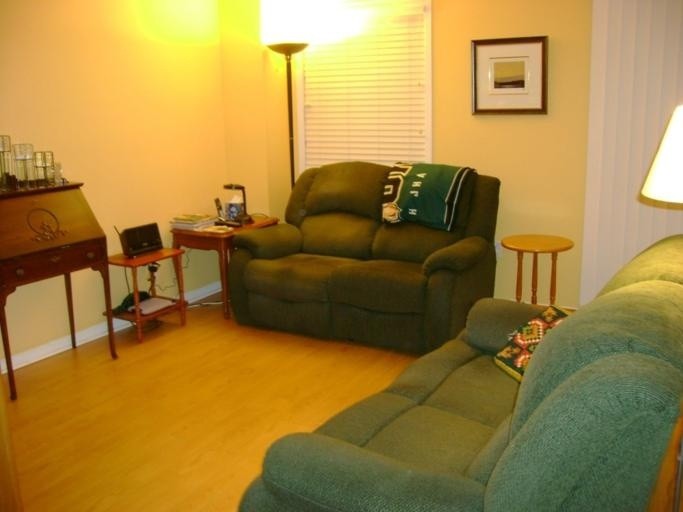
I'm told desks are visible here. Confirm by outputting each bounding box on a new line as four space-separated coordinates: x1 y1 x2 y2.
170 216 278 319
501 233 574 306
0 179 118 401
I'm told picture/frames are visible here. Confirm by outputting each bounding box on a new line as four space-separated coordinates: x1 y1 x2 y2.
467 33 550 117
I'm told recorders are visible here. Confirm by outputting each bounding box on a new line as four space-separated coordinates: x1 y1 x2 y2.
120 222 163 255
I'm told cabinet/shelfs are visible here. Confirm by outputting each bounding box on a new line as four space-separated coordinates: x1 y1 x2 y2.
104 246 189 343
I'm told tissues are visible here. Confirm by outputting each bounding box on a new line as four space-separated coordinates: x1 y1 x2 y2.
223 195 244 220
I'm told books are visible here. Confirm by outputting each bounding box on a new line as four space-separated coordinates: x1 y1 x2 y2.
168 212 218 231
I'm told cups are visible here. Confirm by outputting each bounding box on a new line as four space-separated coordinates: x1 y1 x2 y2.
0 134 63 189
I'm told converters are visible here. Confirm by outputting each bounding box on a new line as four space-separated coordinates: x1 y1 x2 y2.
149 266 157 272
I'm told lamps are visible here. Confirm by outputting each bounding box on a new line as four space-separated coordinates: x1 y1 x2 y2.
261 5 311 191
637 103 683 207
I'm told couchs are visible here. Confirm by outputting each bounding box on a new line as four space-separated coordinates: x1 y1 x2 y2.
224 159 501 359
237 232 682 510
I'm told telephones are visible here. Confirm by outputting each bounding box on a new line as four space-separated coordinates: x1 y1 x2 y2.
214 198 242 227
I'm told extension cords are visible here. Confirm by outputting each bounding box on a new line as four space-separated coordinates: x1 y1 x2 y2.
153 261 159 277
